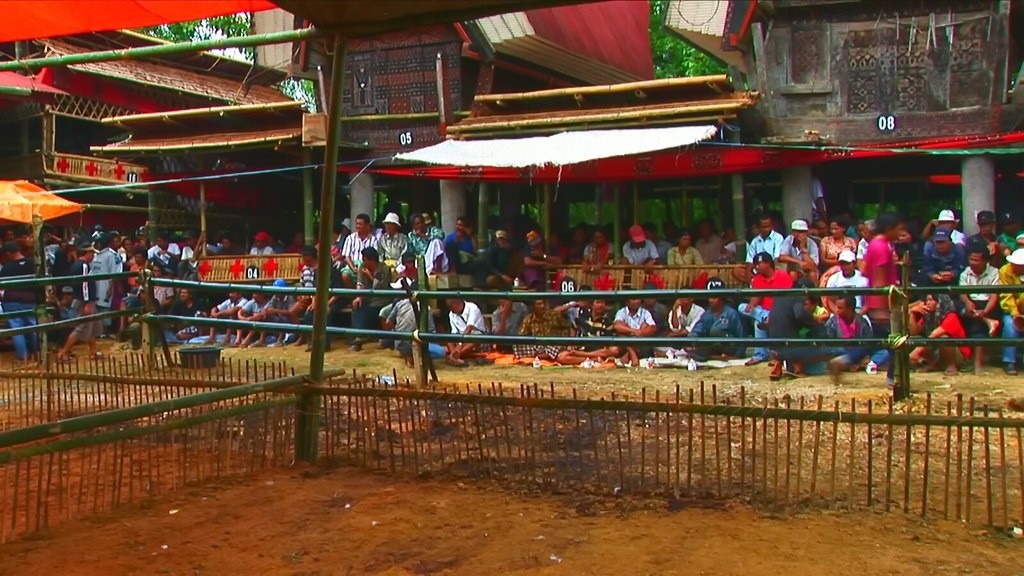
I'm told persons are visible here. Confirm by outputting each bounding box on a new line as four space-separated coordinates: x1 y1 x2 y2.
0 207 1024 389
812 170 828 221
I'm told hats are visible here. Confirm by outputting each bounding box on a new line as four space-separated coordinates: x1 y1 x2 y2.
62 286 73 293
977 210 994 225
791 220 808 231
934 227 948 241
422 213 432 224
382 212 402 228
341 217 350 229
526 231 538 243
838 251 856 263
495 230 507 239
81 245 100 253
629 226 646 243
937 209 955 222
1006 247 1023 265
254 232 271 242
1001 213 1018 224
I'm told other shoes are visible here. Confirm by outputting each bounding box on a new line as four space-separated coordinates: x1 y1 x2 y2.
348 344 360 352
98 333 110 340
1006 363 1016 376
744 359 762 366
758 321 769 329
375 342 390 349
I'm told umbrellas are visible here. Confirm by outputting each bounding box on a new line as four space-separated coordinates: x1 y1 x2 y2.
1 180 87 226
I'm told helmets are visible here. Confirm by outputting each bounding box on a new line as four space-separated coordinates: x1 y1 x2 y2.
272 280 287 287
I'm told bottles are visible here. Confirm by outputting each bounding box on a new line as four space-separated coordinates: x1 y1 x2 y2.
646 357 654 369
688 358 696 370
584 357 591 368
533 357 540 367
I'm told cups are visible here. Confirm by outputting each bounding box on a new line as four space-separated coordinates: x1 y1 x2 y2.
865 360 877 374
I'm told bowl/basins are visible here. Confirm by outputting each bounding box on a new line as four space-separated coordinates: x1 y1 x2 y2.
788 362 826 374
175 346 224 368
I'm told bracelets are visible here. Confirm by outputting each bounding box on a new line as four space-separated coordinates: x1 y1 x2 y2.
458 342 463 346
542 253 547 260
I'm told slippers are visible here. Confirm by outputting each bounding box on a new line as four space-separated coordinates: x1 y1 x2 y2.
782 371 806 378
199 336 314 353
769 372 782 378
55 346 104 364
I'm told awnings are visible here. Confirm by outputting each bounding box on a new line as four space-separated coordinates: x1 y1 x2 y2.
392 125 718 169
